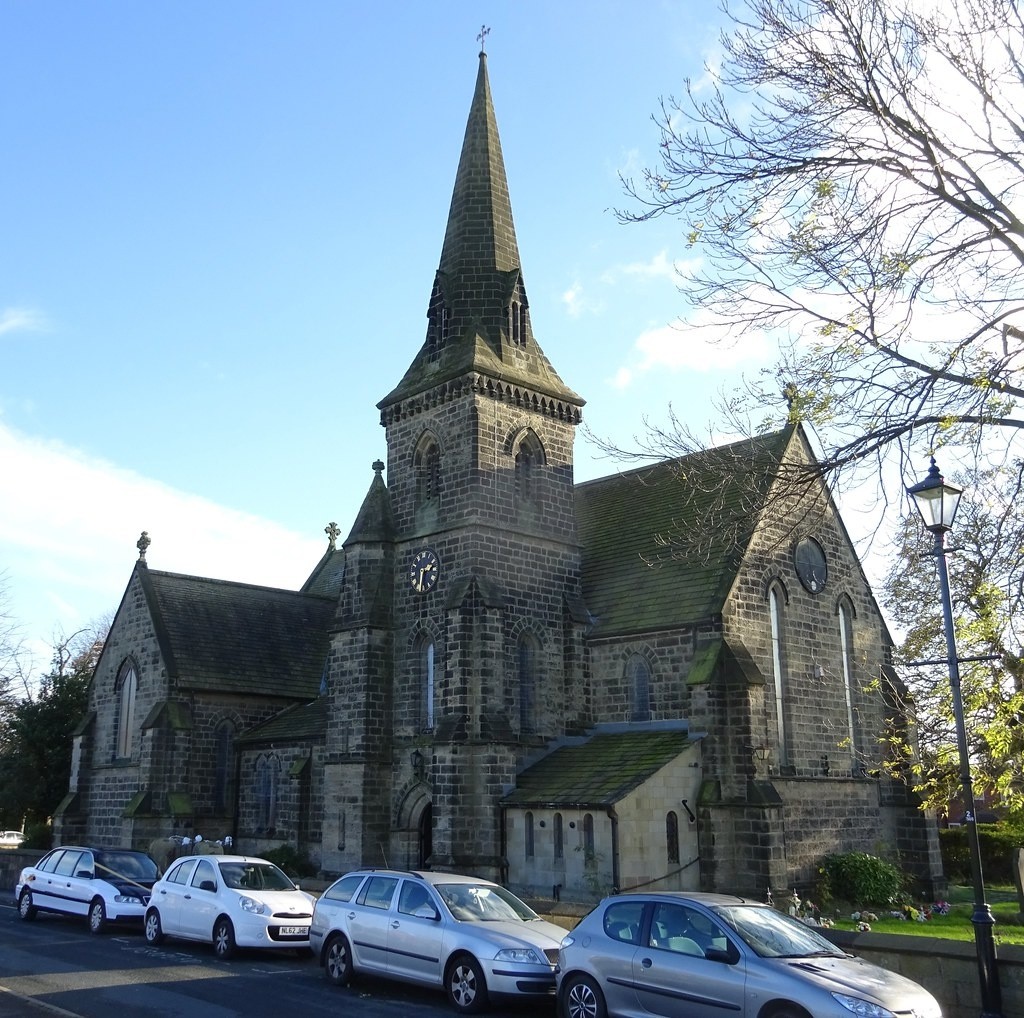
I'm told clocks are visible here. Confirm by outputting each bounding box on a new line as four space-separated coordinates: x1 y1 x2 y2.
408 547 440 595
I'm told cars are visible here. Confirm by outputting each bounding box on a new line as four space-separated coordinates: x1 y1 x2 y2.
0 831 30 845
309 867 571 1015
15 845 163 934
144 854 317 960
554 891 942 1018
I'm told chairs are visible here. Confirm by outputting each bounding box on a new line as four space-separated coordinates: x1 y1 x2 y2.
709 915 729 952
610 921 633 941
405 887 431 916
651 921 668 941
664 936 706 957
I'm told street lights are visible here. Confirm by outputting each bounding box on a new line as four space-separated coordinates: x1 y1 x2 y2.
906 455 1009 1018
